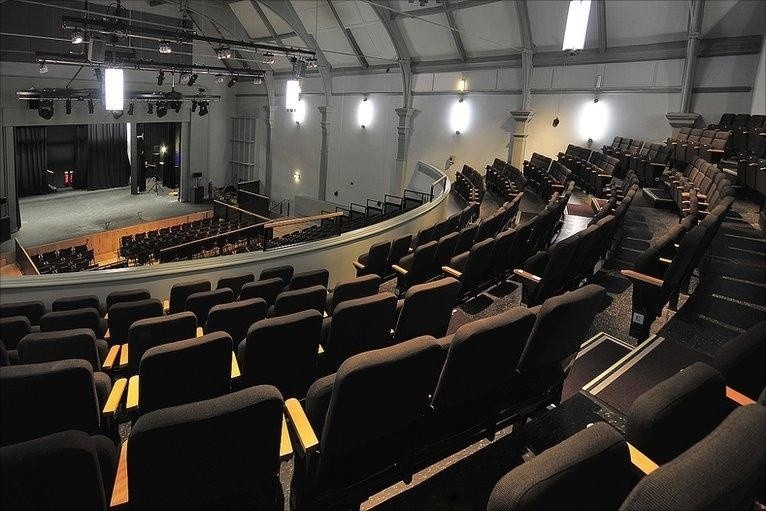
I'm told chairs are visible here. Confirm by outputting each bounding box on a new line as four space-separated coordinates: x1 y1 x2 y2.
0 112 766 511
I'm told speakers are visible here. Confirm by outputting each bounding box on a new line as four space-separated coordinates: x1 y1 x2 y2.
0 217 11 242
191 185 204 204
153 153 160 165
296 60 306 79
88 38 107 63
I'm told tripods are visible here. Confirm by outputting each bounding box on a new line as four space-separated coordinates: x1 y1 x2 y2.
148 166 164 195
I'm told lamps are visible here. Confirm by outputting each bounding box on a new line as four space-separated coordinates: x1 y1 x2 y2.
456 72 468 103
15 1 320 119
292 100 307 128
592 74 603 104
358 98 372 129
